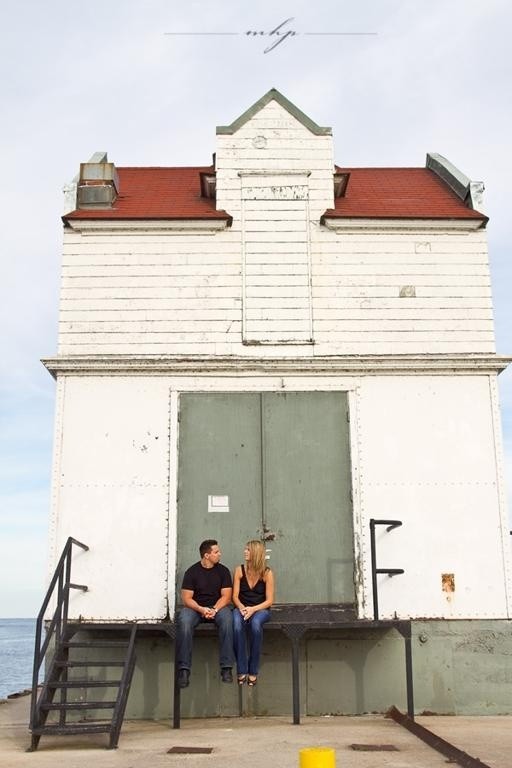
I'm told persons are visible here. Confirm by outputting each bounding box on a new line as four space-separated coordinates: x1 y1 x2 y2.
231 540 275 688
174 539 235 688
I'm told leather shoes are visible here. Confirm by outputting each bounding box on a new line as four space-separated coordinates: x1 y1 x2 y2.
175 667 191 688
219 665 235 684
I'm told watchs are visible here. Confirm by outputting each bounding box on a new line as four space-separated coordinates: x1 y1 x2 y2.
211 606 218 613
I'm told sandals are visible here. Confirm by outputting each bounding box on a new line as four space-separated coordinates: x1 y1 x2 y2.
237 672 259 686
